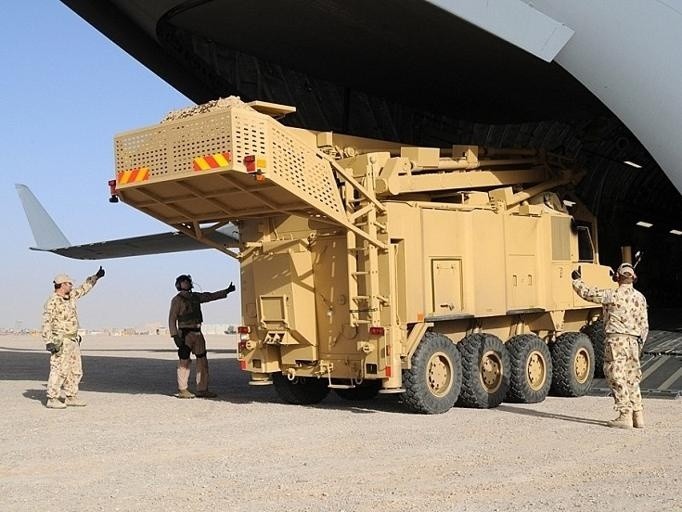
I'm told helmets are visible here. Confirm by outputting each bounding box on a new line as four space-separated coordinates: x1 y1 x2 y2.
175 275 192 291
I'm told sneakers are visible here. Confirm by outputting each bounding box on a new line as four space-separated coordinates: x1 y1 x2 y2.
46 398 67 409
179 389 195 398
633 411 645 428
65 397 88 406
195 390 218 398
606 412 634 429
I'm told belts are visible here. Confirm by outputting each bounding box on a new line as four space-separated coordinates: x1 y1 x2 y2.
53 332 78 338
179 327 200 332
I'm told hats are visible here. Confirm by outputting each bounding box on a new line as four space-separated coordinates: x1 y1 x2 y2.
53 273 75 285
617 262 636 278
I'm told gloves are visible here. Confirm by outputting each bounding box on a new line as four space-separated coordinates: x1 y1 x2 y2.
571 265 582 280
174 335 185 348
95 265 105 278
46 343 59 354
227 282 235 292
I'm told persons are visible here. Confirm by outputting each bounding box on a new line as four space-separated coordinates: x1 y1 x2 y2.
42 266 105 408
571 262 649 430
169 274 235 398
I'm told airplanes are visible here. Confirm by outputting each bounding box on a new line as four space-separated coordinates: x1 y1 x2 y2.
0 0 681 396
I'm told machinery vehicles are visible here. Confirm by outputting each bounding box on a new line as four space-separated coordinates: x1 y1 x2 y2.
109 87 619 414
